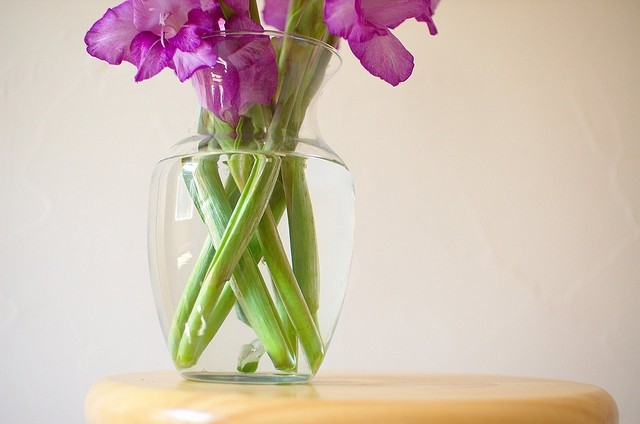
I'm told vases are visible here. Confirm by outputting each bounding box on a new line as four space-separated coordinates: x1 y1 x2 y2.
145 30 362 385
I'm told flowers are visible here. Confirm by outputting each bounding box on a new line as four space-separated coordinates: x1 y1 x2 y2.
84 0 441 372
